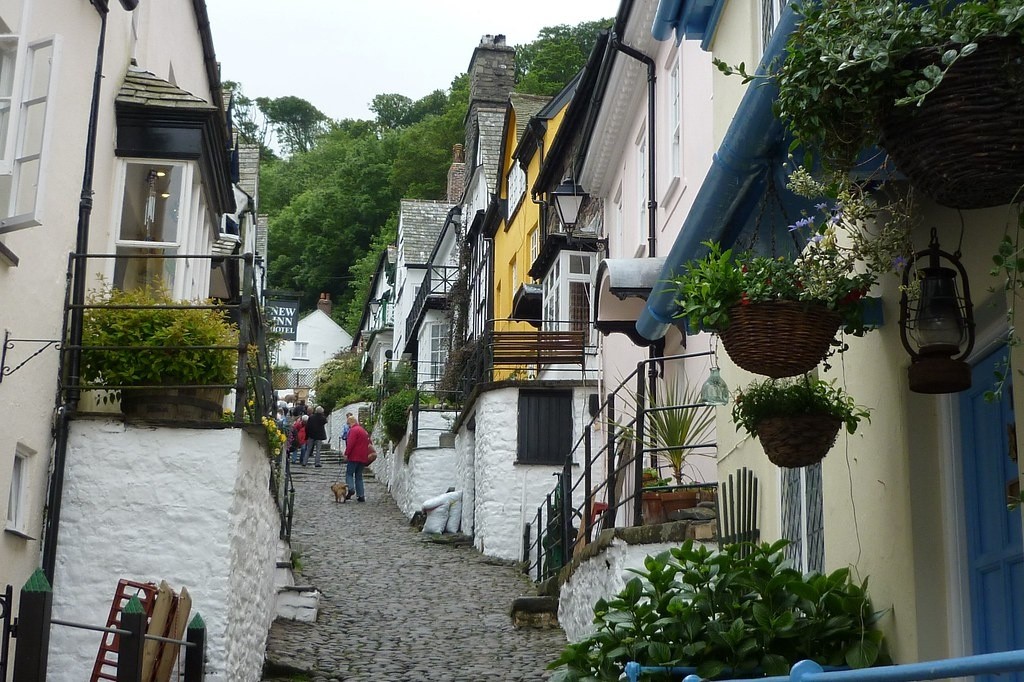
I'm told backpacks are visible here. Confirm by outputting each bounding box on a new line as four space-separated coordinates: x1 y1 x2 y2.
342 425 347 440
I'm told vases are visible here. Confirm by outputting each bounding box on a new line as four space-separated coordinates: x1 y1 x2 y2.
718 298 845 378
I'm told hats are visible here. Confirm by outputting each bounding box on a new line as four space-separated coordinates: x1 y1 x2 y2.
316 406 324 413
302 415 309 420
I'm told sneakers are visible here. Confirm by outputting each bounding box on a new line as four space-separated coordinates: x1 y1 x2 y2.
345 490 355 500
357 496 365 502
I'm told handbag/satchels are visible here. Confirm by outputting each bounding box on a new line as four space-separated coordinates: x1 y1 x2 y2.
366 432 377 467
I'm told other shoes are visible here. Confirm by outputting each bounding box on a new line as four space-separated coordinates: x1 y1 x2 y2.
315 464 322 467
302 463 312 466
292 460 296 464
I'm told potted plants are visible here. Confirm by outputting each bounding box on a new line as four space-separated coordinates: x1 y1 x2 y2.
596 366 716 526
727 371 875 468
65 271 258 423
711 0 1024 209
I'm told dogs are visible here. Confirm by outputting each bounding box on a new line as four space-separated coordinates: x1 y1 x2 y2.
330 481 350 504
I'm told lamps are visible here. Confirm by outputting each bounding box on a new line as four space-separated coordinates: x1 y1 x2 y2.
898 194 977 395
701 332 729 406
549 175 610 252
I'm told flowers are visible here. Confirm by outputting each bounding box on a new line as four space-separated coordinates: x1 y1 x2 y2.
666 152 925 339
219 401 287 460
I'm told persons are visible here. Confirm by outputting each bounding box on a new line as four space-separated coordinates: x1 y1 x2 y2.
278 398 316 458
342 413 353 448
292 414 309 464
342 416 376 503
302 406 328 467
